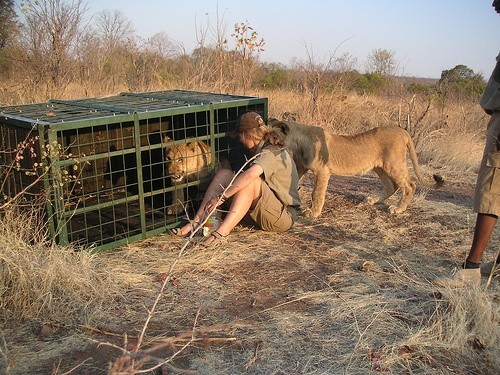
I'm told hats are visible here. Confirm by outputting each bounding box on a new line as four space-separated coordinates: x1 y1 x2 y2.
230 112 265 136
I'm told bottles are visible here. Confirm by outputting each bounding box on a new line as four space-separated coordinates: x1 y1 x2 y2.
203 207 222 237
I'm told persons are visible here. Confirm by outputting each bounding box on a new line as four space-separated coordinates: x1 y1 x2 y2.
460 0 500 276
169 112 302 250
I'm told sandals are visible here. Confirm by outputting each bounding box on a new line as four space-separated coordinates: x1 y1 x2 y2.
199 231 230 250
167 222 202 238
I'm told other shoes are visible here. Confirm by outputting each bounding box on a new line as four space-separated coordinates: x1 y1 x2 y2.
434 265 481 287
482 261 500 275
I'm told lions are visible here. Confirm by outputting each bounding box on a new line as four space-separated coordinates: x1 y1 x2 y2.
268 117 445 220
163 137 233 216
